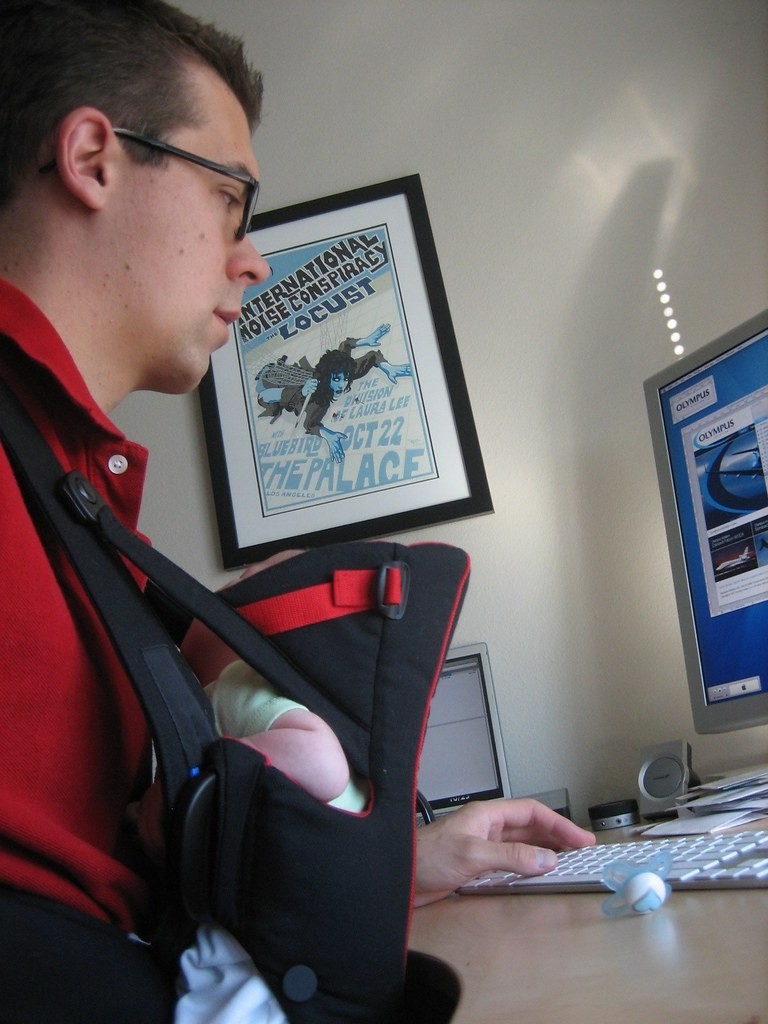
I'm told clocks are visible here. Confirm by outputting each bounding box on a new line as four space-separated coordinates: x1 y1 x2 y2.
637 740 701 822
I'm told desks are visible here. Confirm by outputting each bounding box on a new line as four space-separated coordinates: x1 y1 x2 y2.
407 820 767 1024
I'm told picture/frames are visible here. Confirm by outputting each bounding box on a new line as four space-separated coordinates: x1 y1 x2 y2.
196 174 495 570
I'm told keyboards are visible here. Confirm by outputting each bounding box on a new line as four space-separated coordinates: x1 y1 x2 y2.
455 829 768 897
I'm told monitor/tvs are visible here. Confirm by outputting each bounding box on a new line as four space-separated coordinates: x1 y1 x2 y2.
640 306 768 733
413 641 513 819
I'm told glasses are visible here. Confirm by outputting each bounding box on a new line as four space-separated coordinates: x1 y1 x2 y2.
37 122 259 241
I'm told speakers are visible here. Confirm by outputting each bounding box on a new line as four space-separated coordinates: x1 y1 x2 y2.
638 738 701 820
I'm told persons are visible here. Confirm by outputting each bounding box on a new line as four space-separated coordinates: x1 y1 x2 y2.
0 0 597 1024
127 548 366 1024
